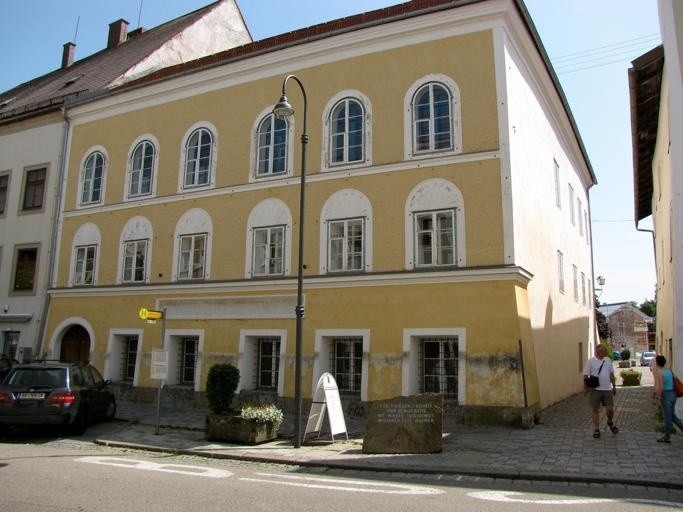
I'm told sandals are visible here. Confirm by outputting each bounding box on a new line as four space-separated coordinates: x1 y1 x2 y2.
593 429 601 438
609 422 619 434
657 436 672 443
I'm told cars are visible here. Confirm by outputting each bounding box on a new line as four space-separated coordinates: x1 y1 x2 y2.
640 352 657 366
0 353 116 437
612 351 622 360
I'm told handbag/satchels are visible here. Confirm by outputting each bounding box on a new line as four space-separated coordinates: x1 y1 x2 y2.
673 376 683 397
586 375 600 389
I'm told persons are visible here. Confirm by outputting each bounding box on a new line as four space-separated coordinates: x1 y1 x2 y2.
583 344 617 438
652 355 682 443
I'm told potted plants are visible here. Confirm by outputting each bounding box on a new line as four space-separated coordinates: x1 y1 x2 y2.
204 364 285 445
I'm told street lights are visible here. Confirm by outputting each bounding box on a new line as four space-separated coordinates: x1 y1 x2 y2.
273 74 309 448
